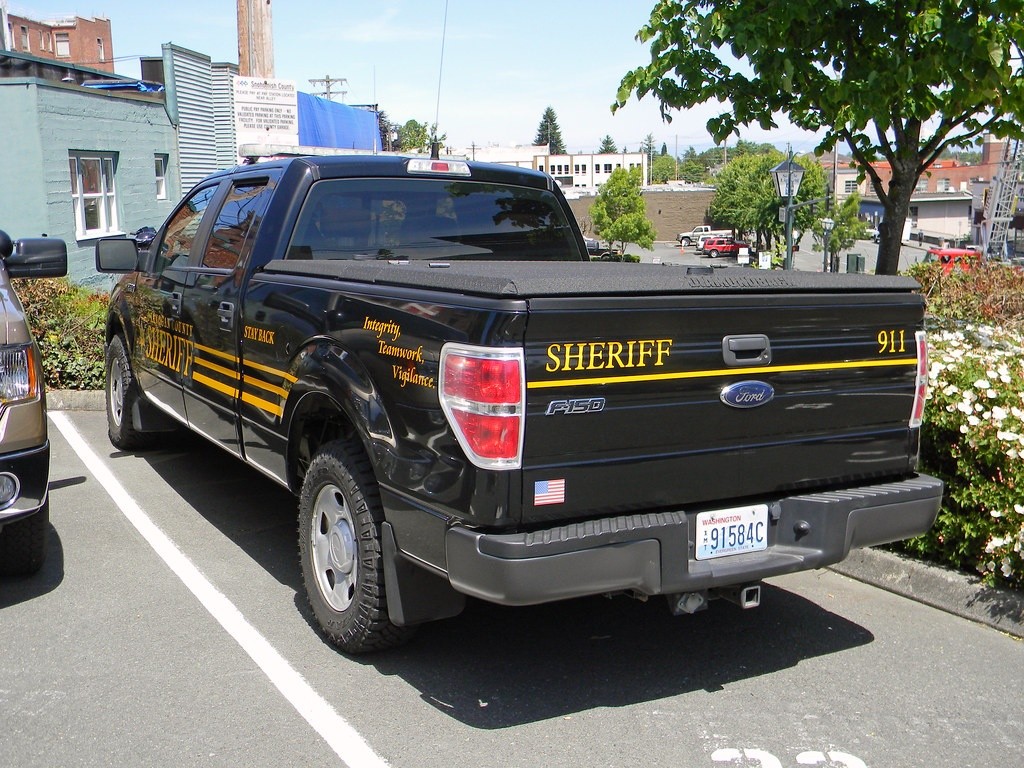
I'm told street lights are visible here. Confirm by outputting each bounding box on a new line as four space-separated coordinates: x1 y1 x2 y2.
770 148 807 270
821 216 836 271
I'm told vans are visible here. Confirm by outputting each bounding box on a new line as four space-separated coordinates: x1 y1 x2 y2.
696 235 732 251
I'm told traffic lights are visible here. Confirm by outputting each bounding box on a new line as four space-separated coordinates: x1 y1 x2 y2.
792 246 799 252
827 187 836 211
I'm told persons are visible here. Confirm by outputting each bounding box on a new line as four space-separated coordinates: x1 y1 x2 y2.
917 229 924 247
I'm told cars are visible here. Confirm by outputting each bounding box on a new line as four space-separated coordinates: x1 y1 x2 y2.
0 231 69 528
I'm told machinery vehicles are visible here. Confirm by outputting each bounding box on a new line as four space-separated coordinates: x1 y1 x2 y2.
922 131 1023 278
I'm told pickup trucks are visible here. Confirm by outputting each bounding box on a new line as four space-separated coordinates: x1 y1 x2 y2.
588 249 623 262
676 226 733 247
94 157 949 660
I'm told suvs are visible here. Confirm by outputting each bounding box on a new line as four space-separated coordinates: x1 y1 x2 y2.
702 237 750 258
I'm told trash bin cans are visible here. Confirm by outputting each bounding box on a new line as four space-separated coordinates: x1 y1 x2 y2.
938 236 945 246
